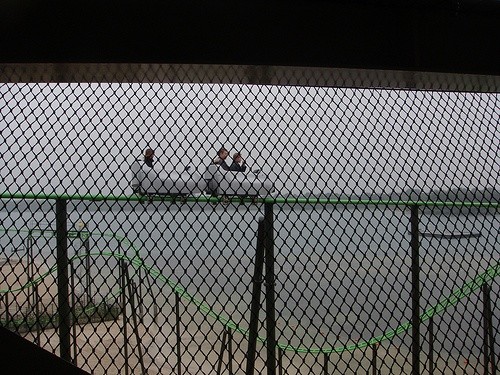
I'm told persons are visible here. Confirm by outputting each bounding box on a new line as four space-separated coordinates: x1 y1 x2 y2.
213 149 230 171
145 148 154 170
229 152 248 173
